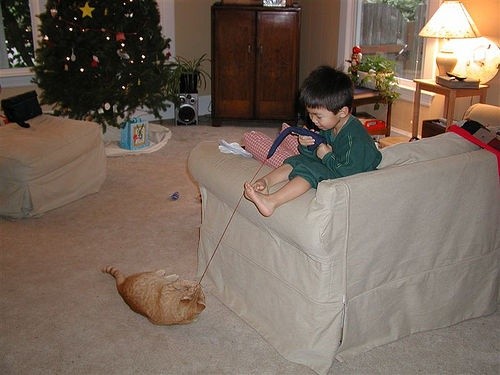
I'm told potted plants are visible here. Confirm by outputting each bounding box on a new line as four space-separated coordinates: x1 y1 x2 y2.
352 54 401 108
172 53 212 93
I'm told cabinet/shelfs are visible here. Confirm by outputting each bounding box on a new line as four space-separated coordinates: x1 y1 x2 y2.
210 3 302 126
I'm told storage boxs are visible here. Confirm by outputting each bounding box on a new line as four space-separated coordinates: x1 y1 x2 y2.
422 117 456 137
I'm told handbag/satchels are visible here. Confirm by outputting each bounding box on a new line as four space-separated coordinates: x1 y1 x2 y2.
0 89 43 128
120 117 149 150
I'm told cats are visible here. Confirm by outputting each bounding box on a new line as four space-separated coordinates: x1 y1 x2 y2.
101 264 206 326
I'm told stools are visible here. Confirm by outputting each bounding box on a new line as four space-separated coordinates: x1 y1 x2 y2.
1 114 109 219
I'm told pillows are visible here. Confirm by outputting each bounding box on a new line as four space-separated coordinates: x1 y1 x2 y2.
277 122 310 156
243 131 292 168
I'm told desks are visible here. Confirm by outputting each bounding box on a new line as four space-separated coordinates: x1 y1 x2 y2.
411 78 488 138
347 74 394 137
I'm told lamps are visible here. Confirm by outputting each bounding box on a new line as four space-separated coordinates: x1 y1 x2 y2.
418 1 482 77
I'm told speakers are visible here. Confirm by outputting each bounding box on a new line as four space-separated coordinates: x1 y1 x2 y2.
174 93 199 126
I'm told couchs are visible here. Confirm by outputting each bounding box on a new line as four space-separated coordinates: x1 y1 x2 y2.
188 104 500 374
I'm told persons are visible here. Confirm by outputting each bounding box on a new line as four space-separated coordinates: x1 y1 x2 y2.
244 65 383 218
